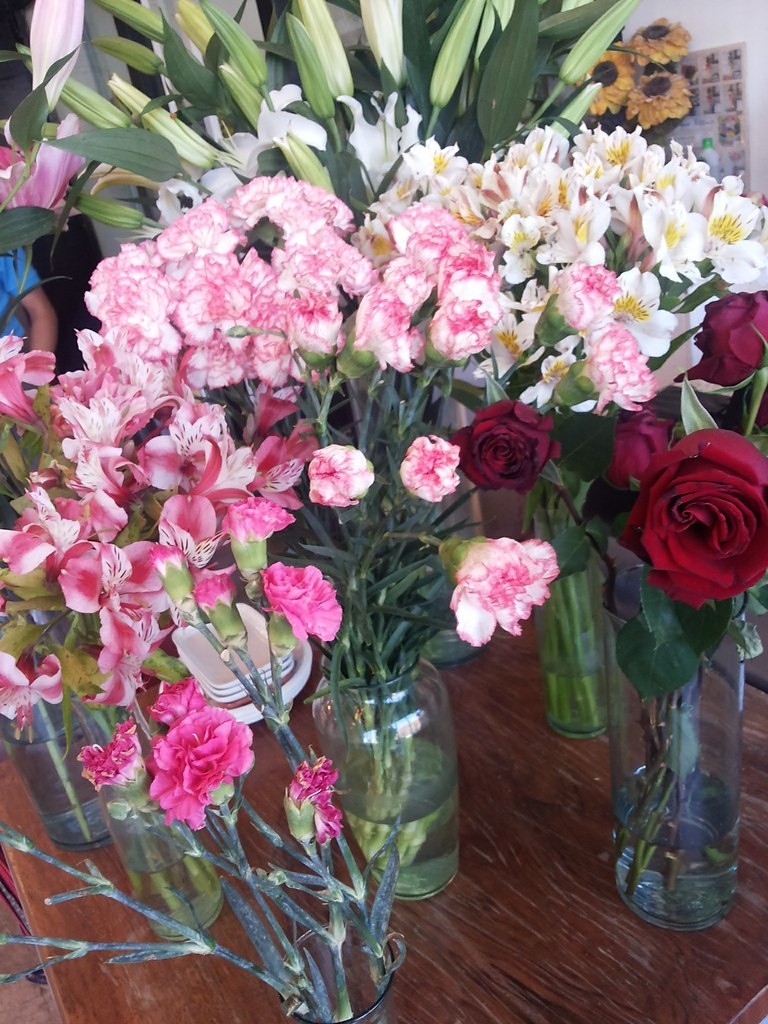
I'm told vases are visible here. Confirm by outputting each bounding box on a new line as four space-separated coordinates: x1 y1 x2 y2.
603 566 743 930
278 922 398 1024
97 772 225 943
333 338 488 665
311 652 461 901
540 555 614 736
3 693 141 851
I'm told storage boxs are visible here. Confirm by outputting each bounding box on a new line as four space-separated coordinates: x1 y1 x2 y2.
170 602 314 725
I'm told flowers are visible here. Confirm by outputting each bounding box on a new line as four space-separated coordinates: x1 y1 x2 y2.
1 0 768 1020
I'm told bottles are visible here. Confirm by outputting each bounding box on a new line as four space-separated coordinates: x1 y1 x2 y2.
699 138 719 182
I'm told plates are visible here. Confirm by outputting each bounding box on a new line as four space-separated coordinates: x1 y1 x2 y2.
172 602 293 709
178 635 313 724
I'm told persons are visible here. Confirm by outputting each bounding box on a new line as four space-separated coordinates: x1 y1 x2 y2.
0 248 60 470
